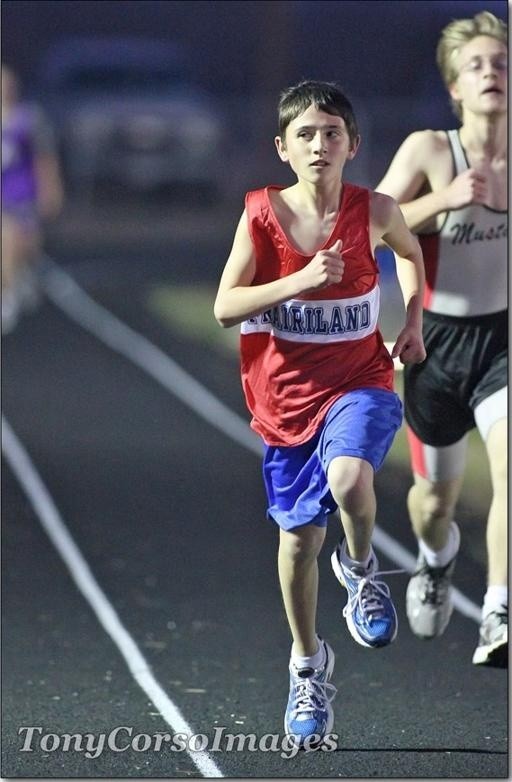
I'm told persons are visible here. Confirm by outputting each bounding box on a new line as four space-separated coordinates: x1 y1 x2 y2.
1 61 65 333
371 10 507 669
209 78 427 752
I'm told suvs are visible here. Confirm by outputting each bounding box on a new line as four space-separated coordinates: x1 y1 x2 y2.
25 29 229 207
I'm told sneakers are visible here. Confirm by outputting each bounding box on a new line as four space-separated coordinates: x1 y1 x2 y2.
472 600 508 674
406 521 461 642
328 534 398 650
283 634 335 749
